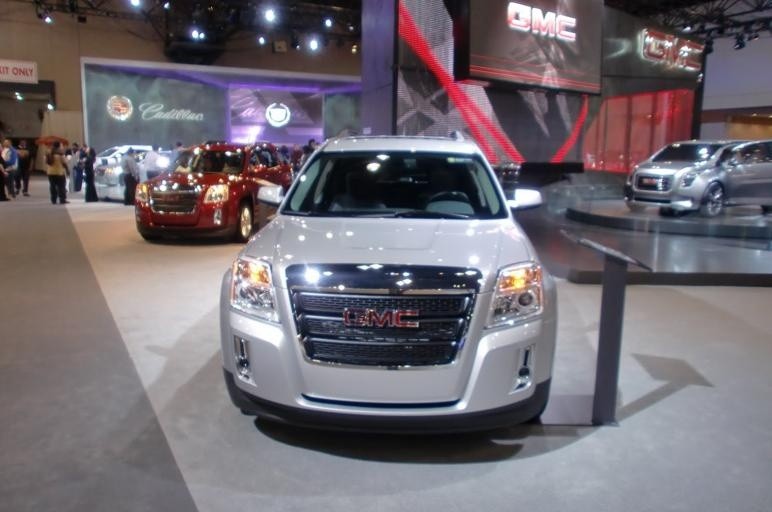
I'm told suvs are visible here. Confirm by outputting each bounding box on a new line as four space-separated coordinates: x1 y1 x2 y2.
219 128 558 436
135 140 294 243
83 145 151 182
623 139 771 219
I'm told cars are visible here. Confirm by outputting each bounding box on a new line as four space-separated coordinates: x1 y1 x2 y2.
94 150 173 186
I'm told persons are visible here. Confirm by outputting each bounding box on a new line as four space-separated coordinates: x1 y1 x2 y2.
1 138 99 205
279 138 318 173
122 142 188 204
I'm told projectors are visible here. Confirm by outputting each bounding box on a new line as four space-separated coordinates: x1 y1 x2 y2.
152 16 225 65
